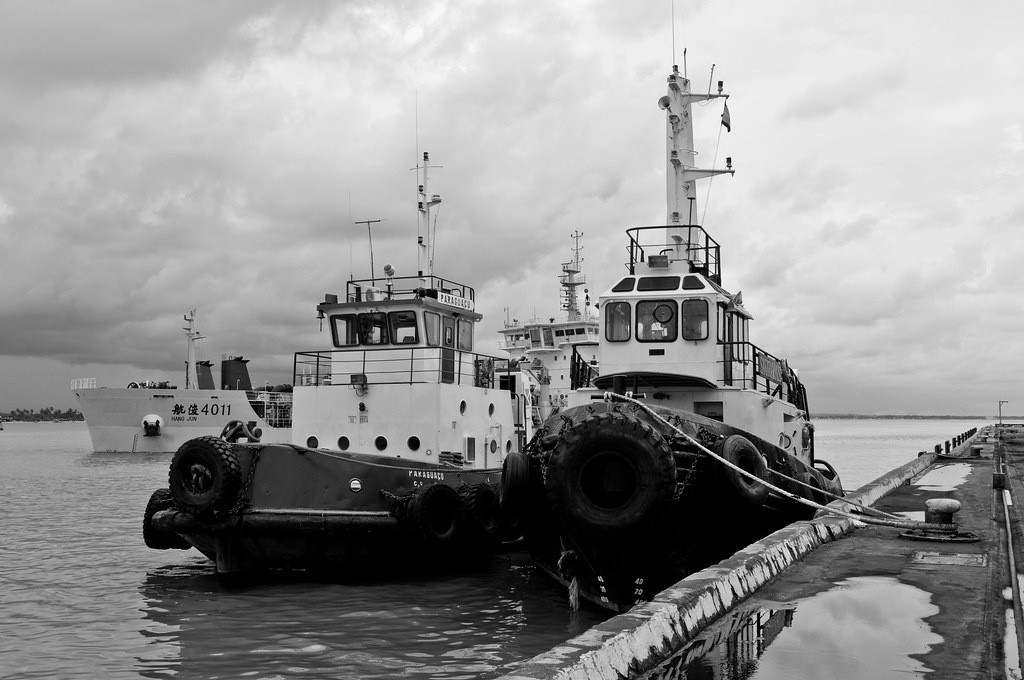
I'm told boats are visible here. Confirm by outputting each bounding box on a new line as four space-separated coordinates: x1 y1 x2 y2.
516 0 843 612
151 108 599 577
69 300 332 454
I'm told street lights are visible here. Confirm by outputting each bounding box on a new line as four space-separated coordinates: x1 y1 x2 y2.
997 398 1009 427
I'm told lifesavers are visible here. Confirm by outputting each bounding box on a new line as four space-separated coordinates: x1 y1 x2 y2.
412 483 464 545
545 412 681 542
466 481 498 543
720 435 770 503
496 451 532 521
803 424 810 453
167 434 243 514
794 470 828 507
142 487 192 552
